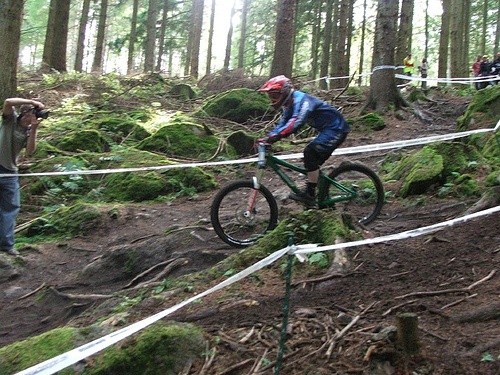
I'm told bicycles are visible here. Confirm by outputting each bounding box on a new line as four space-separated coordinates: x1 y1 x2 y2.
210 136 385 248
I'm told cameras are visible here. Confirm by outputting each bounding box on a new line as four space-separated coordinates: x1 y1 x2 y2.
32 108 50 120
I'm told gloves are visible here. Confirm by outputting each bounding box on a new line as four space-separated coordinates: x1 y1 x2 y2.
269 133 279 143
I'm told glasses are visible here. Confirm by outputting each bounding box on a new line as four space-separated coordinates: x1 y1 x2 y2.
268 91 281 102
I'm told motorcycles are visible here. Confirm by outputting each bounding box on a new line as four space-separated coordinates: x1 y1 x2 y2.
475 62 500 90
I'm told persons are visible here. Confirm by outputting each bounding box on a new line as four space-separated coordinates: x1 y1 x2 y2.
244 75 354 205
0 92 48 256
471 55 496 87
418 59 427 89
404 53 414 78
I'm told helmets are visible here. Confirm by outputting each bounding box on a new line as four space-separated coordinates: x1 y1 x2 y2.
257 75 294 111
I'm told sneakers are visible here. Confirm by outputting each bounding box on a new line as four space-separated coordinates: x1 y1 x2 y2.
289 192 315 206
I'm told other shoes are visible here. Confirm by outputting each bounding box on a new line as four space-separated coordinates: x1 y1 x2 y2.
6 249 20 255
